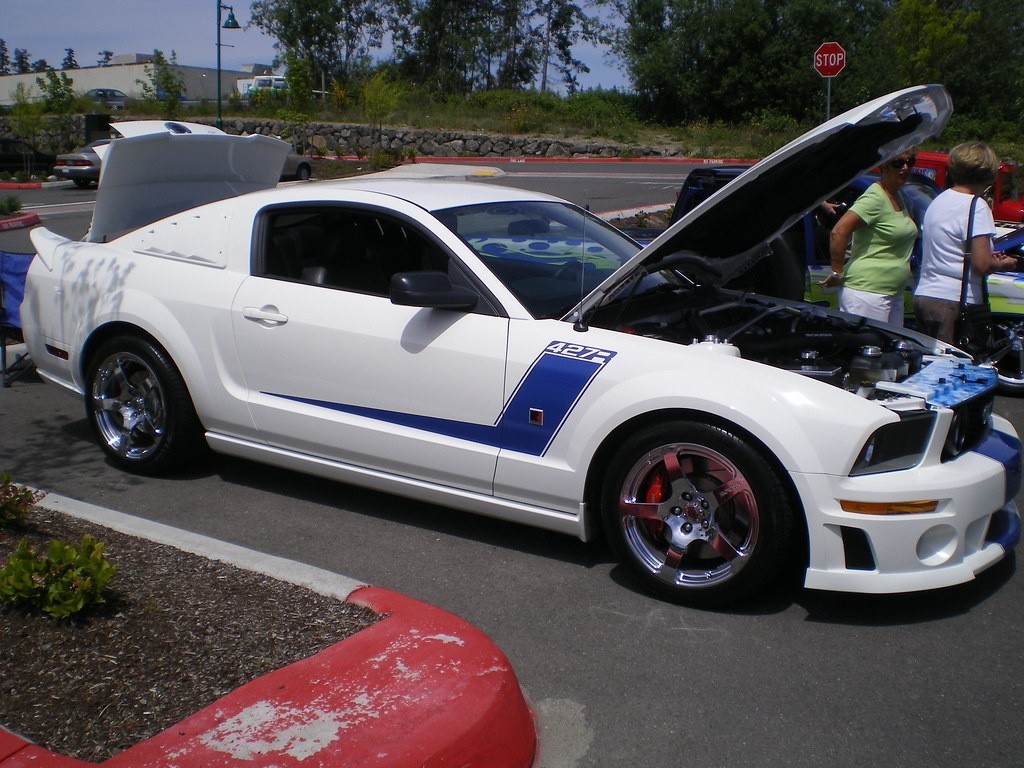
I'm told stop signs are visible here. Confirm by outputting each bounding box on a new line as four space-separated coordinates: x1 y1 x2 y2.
813 42 846 78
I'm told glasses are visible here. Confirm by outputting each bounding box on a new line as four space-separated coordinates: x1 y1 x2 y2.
887 154 915 169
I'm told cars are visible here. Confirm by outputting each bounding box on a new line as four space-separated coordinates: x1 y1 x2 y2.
76 89 130 112
447 162 1024 391
0 138 57 181
53 138 116 190
874 152 1024 222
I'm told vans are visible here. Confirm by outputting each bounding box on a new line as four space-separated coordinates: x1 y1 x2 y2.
249 76 311 94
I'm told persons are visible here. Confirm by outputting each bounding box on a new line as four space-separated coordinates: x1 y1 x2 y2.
912 139 1017 347
782 200 840 309
817 149 918 328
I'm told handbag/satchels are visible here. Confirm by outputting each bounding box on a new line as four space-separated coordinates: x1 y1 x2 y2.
953 303 996 355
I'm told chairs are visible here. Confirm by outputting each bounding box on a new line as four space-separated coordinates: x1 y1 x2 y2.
303 219 390 293
412 213 475 290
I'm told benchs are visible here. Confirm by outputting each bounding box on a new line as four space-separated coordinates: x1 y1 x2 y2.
272 225 328 277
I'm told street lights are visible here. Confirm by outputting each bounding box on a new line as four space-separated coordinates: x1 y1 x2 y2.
216 0 241 134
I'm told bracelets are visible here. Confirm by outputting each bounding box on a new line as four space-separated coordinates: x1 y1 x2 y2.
831 270 844 279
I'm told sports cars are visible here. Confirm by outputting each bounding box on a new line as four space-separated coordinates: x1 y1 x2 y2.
15 83 1024 608
